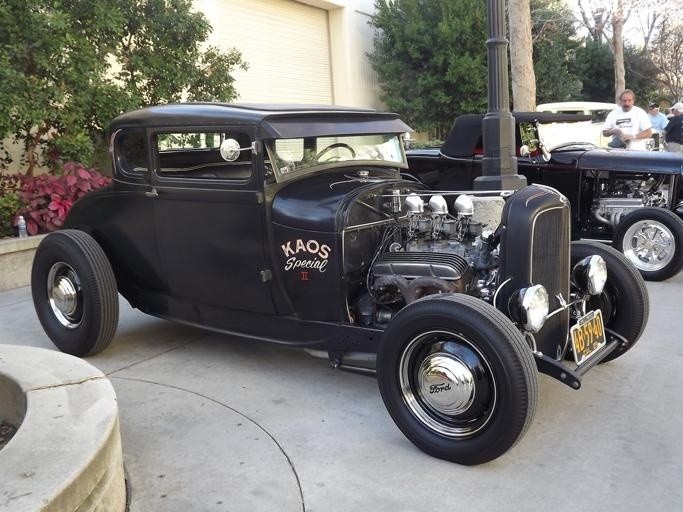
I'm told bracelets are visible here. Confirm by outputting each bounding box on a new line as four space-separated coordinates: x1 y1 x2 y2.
633 135 636 140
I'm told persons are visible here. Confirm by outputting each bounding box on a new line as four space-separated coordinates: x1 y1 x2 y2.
602 88 651 151
647 102 683 152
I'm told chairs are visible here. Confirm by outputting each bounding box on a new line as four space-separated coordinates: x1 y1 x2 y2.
128 146 273 179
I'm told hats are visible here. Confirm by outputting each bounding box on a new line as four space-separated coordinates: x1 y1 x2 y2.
648 103 659 111
670 102 683 110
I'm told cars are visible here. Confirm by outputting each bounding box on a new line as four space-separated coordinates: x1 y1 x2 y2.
535 101 661 152
399 110 682 281
29 103 650 466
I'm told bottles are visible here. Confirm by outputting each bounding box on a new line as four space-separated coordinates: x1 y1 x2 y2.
18 216 27 238
